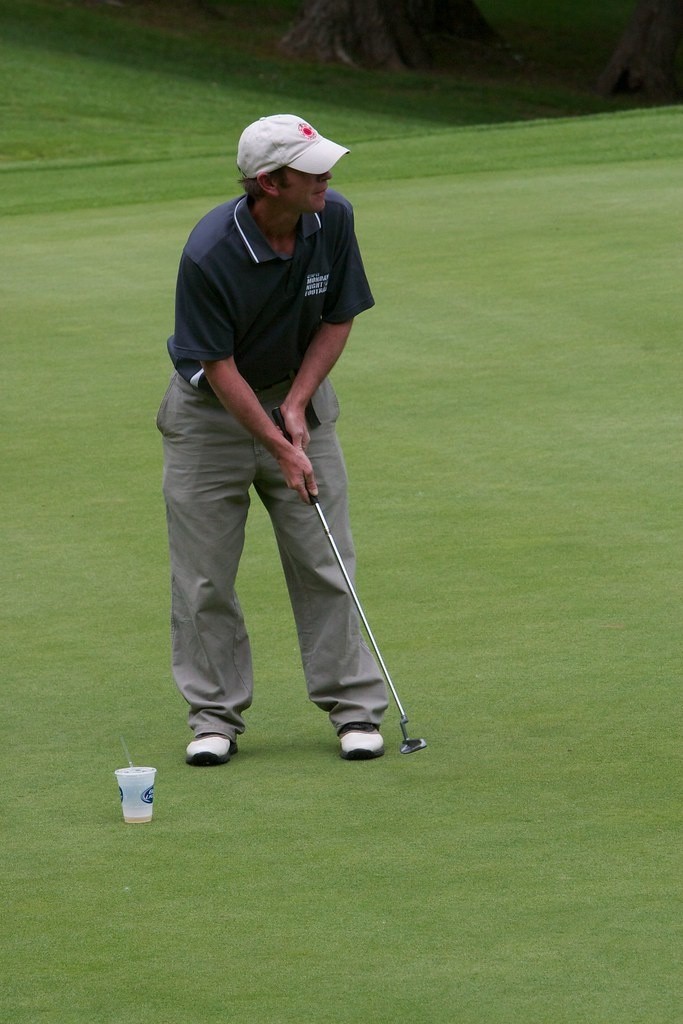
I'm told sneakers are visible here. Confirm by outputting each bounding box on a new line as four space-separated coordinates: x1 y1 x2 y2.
184 735 241 768
338 725 387 760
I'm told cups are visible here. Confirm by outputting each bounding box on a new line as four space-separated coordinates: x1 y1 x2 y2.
115 768 156 823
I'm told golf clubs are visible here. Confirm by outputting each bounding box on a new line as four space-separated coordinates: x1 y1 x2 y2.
271 406 428 755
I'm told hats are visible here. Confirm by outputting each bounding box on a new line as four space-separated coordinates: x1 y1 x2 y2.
238 114 352 180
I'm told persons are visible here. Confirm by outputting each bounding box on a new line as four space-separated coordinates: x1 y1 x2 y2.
156 113 389 766
